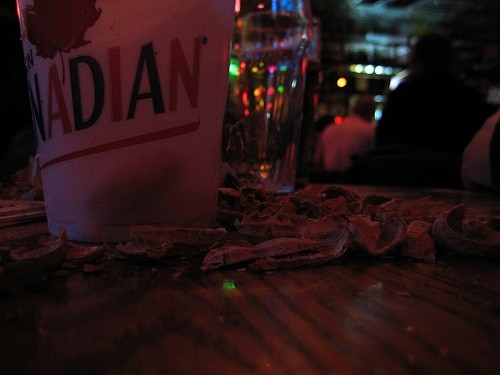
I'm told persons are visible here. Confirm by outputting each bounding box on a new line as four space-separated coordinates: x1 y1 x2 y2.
372 32 479 185
311 104 375 187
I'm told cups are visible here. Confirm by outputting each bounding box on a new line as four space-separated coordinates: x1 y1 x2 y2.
233 0 315 194
12 0 236 242
